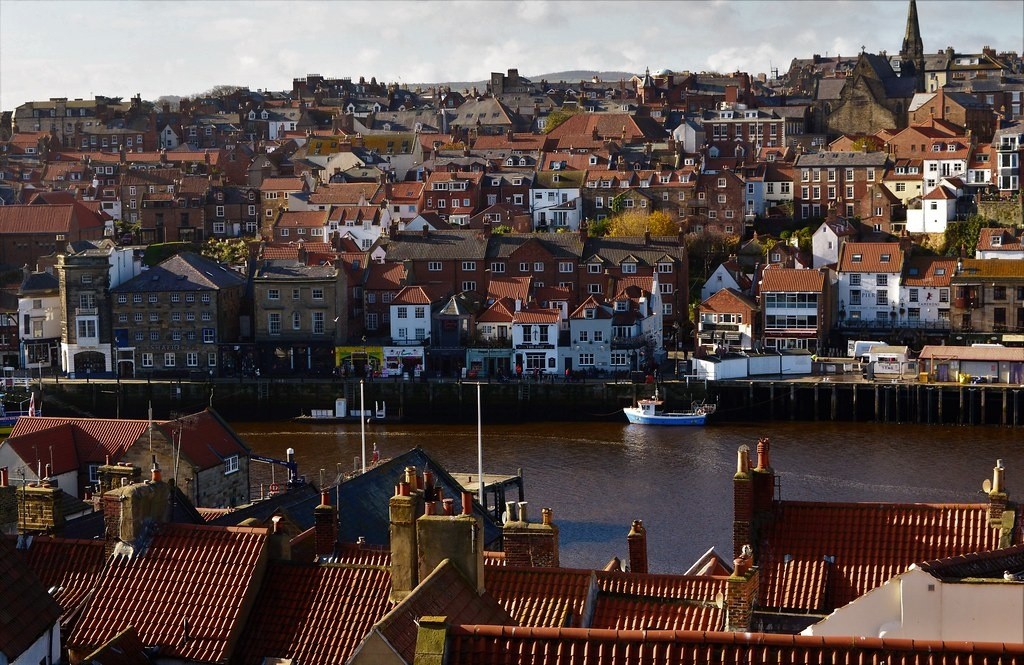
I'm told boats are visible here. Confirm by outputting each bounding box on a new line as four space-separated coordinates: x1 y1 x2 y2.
0 360 44 440
623 387 717 426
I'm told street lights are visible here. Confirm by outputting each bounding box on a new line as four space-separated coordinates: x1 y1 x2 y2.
613 336 619 385
115 338 120 378
487 335 494 384
7 312 22 373
673 321 680 381
361 335 368 382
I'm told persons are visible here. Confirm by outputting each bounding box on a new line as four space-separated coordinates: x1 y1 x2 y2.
452 361 578 383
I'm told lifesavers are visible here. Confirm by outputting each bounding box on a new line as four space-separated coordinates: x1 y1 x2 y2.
270 483 280 494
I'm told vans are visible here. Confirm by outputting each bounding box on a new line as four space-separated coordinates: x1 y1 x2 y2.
847 339 889 359
972 343 1005 347
